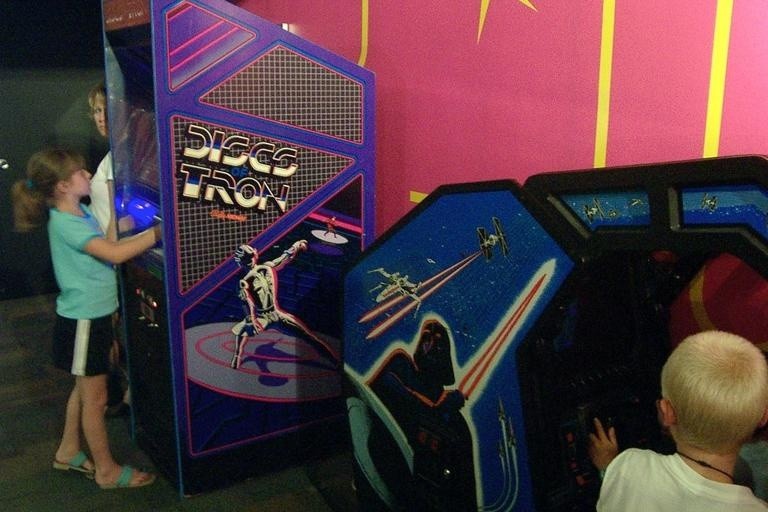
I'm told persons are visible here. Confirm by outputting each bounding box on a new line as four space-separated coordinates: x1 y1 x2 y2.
586 330 766 511
24 145 163 492
84 80 131 419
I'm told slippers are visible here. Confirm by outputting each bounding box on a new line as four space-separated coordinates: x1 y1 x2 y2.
52 449 95 473
99 464 156 489
